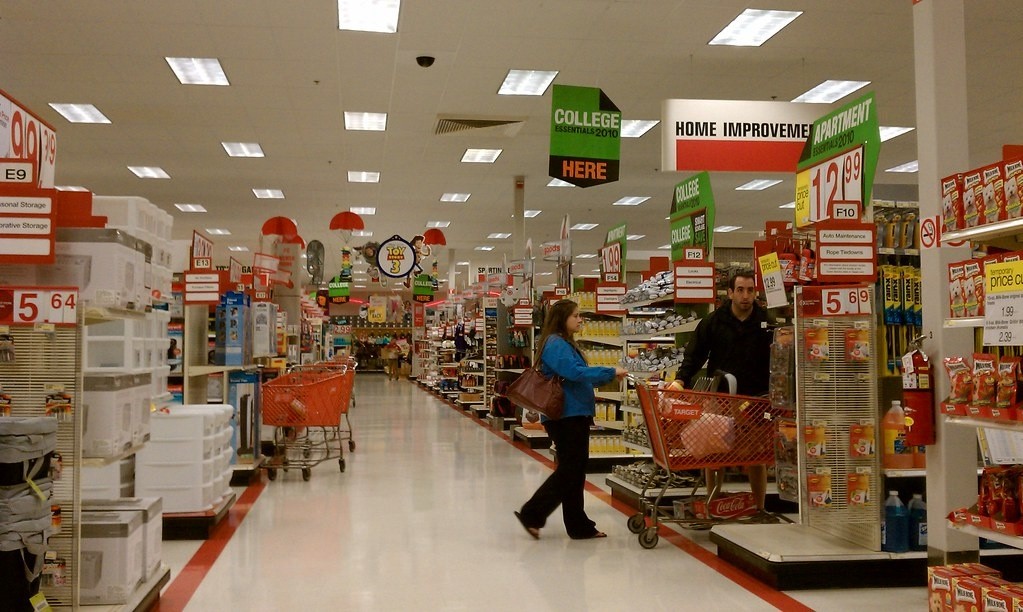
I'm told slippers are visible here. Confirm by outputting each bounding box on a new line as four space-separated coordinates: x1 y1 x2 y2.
513 511 540 538
571 529 607 540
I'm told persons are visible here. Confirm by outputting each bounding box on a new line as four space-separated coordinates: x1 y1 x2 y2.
666 271 771 515
386 338 402 382
515 300 629 541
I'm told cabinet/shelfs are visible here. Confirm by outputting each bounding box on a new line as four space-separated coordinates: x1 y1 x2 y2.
1 227 288 612
939 218 1022 551
414 200 921 590
167 283 266 475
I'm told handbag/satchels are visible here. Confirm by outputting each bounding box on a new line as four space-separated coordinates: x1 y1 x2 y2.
505 334 567 416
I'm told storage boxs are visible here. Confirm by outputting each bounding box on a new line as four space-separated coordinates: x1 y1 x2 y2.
0 194 240 612
927 145 1023 612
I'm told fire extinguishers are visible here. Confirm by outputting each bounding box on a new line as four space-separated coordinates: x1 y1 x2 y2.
901 335 935 445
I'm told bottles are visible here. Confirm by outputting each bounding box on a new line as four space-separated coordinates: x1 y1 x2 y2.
880 491 927 553
577 321 623 338
579 347 621 368
563 290 599 310
878 401 927 470
588 387 626 455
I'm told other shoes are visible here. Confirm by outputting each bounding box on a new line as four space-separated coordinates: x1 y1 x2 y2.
751 510 780 523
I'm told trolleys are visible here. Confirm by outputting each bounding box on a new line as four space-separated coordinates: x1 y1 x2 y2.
258 355 358 482
621 371 780 549
